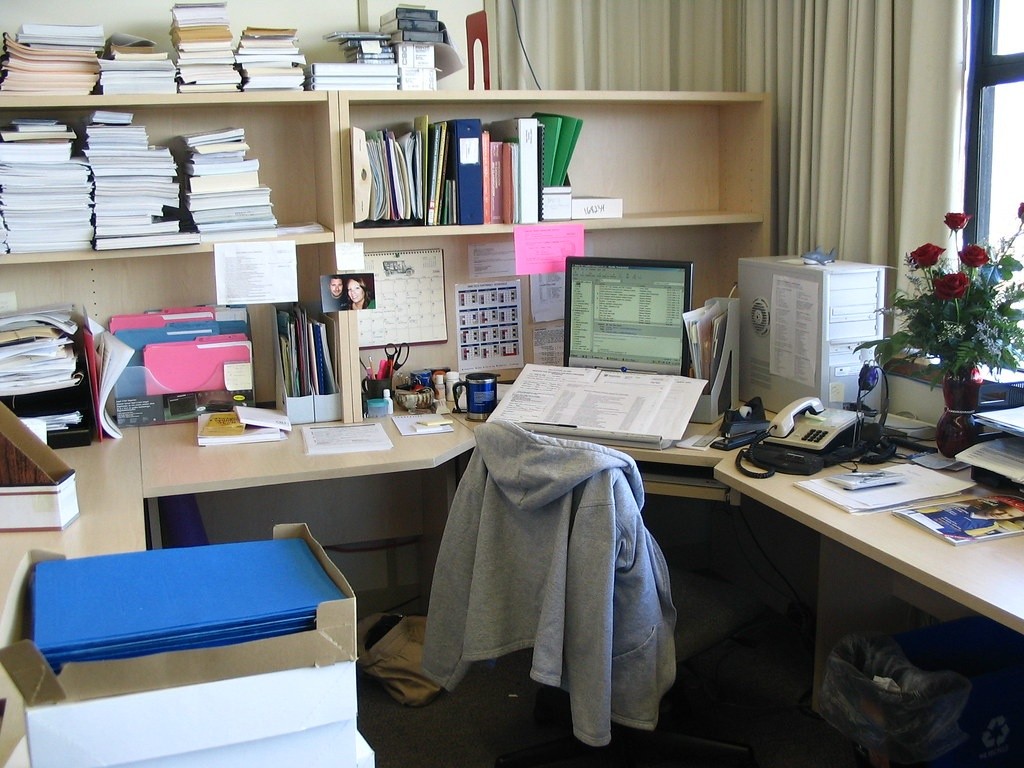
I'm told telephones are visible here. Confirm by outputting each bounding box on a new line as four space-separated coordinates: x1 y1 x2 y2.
763 396 864 455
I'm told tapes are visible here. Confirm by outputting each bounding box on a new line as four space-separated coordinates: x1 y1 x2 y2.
739 406 752 419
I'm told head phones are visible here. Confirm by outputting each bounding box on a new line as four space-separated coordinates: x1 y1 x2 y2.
857 363 890 442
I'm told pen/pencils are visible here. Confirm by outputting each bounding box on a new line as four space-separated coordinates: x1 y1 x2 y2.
369 357 375 380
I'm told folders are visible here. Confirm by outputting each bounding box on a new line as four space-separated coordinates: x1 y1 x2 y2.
531 110 582 187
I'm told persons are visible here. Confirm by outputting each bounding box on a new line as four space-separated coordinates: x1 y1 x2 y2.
912 497 1024 540
322 274 375 312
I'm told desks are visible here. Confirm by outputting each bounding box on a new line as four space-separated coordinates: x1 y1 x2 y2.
136 411 475 553
450 379 778 507
0 419 148 705
715 416 1024 722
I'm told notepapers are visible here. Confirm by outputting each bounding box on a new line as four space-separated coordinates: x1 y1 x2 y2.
416 419 453 426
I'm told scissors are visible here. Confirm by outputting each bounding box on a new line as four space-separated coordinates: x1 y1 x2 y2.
385 343 409 378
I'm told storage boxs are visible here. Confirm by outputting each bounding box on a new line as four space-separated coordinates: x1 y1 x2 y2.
0 399 78 481
0 515 361 768
0 472 83 533
322 8 445 91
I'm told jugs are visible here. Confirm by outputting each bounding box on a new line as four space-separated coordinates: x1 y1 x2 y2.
453 371 501 420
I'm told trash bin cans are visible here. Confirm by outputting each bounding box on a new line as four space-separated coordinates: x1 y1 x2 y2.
828 615 1024 768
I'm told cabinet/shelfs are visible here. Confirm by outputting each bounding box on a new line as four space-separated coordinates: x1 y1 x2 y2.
340 85 779 413
0 86 352 421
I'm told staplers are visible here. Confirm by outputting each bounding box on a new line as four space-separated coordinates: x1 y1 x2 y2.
710 420 771 451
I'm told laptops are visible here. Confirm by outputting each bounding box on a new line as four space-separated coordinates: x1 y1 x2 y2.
562 255 694 378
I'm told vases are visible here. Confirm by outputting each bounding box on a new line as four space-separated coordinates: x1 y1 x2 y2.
935 361 985 460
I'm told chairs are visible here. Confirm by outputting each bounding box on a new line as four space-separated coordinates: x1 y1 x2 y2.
467 426 767 768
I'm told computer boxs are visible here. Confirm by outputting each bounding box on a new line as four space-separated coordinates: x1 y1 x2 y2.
738 256 885 423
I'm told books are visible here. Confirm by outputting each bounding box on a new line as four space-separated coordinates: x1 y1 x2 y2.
891 494 1024 547
350 112 584 229
0 110 278 256
0 0 465 95
391 413 454 435
197 405 291 447
825 469 904 490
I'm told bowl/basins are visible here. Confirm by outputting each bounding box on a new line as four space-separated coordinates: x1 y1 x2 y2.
395 391 434 411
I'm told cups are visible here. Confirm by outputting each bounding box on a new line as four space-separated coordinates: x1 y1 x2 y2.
366 399 388 417
362 374 392 400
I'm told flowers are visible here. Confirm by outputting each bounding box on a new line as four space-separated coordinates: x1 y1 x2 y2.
847 197 1024 393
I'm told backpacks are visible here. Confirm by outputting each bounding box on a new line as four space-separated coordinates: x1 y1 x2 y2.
356 611 442 709
